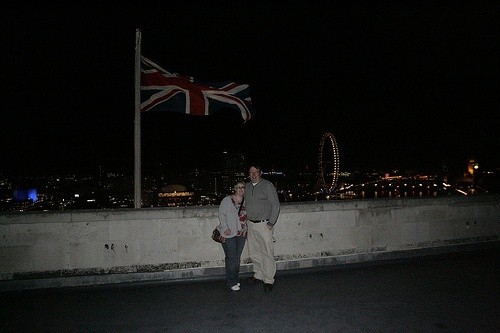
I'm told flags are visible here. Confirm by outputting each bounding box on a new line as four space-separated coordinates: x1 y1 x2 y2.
139 52 255 127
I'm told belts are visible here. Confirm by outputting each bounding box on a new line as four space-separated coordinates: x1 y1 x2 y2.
249 219 269 223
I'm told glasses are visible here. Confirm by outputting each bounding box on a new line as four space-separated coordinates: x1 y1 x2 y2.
235 186 245 190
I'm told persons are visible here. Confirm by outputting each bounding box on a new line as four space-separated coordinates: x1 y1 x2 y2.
216 181 248 291
245 164 280 292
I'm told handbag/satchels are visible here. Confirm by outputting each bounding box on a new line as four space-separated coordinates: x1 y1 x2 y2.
211 229 225 244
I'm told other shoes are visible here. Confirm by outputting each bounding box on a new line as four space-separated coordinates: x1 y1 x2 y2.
264 284 275 292
245 275 261 285
230 282 241 291
